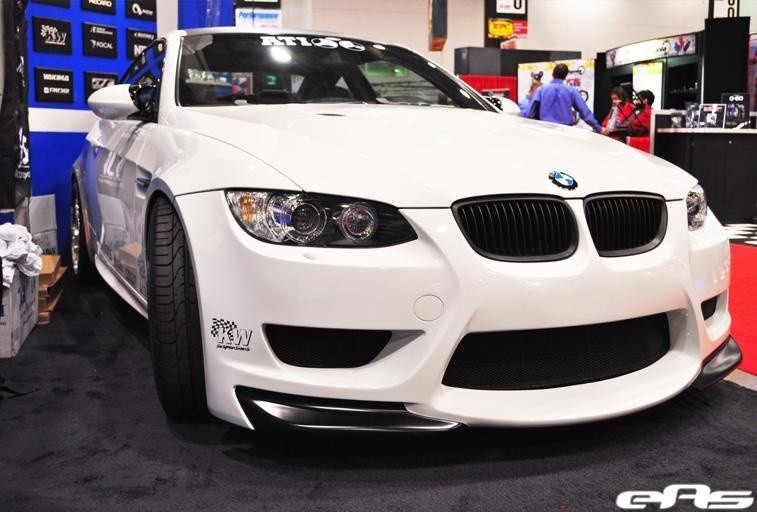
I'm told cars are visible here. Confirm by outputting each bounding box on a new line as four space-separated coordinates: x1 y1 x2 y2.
70 25 740 444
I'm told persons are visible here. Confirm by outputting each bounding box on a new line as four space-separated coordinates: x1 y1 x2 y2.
524 63 610 136
518 81 544 119
616 90 654 153
600 87 637 144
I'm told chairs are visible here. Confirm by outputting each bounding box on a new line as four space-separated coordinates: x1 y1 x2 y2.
183 80 355 105
